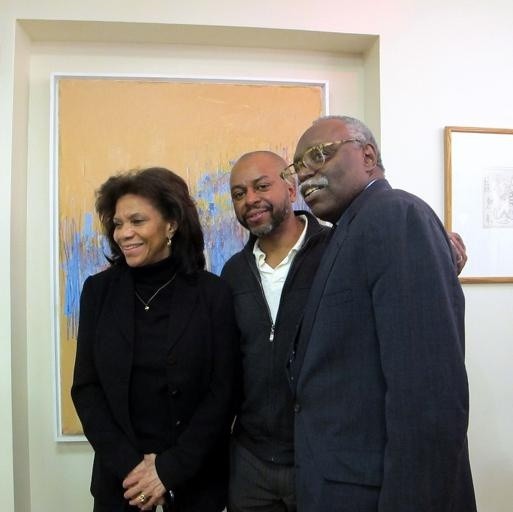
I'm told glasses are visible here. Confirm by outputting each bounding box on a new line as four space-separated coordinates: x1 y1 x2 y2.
279 138 368 185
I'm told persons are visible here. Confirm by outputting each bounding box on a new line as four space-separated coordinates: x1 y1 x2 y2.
284 116 478 509
215 150 469 510
70 168 244 511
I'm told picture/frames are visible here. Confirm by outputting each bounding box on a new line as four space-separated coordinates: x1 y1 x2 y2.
442 125 513 285
49 69 330 445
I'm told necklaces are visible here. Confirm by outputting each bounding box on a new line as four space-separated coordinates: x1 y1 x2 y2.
135 268 177 312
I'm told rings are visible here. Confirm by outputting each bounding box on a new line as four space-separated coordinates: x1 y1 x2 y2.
136 493 147 503
456 255 463 262
